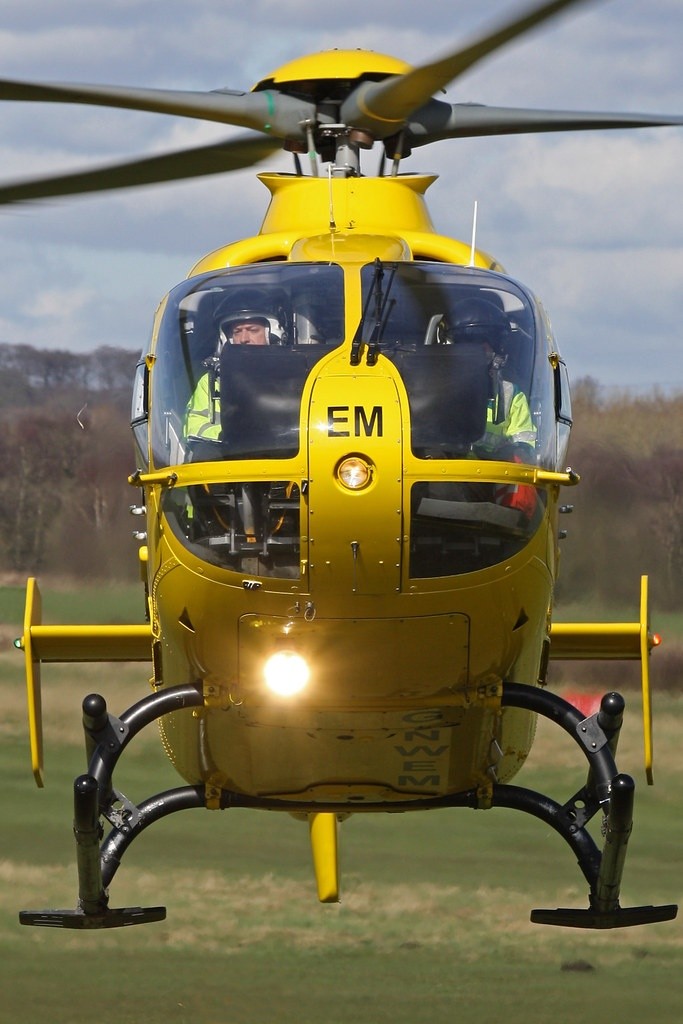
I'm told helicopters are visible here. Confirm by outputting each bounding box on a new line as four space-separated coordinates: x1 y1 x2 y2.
0 0 683 930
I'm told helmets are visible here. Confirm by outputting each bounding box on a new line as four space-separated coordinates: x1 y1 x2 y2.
217 300 288 346
435 296 512 343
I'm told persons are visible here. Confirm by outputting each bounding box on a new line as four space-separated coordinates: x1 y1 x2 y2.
437 298 539 518
182 289 289 520
